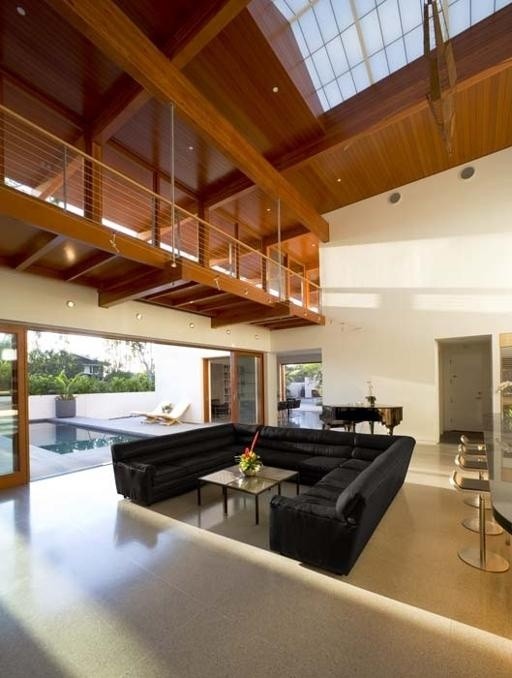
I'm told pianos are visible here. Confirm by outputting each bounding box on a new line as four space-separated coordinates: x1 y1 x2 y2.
319 404 403 436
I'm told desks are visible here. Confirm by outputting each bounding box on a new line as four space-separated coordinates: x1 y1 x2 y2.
196 464 300 526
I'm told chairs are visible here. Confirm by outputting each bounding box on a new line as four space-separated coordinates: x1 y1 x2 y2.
318 405 356 433
212 398 229 418
129 397 191 426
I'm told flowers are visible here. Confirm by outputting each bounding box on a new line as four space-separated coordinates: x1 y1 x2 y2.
235 449 263 475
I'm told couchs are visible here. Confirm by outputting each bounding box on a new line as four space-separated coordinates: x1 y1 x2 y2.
111 422 264 506
269 435 415 576
252 425 356 486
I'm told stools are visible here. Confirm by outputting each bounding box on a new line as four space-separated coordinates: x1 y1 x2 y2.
451 433 510 573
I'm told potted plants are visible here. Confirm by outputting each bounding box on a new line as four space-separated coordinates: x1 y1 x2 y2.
52 368 90 418
